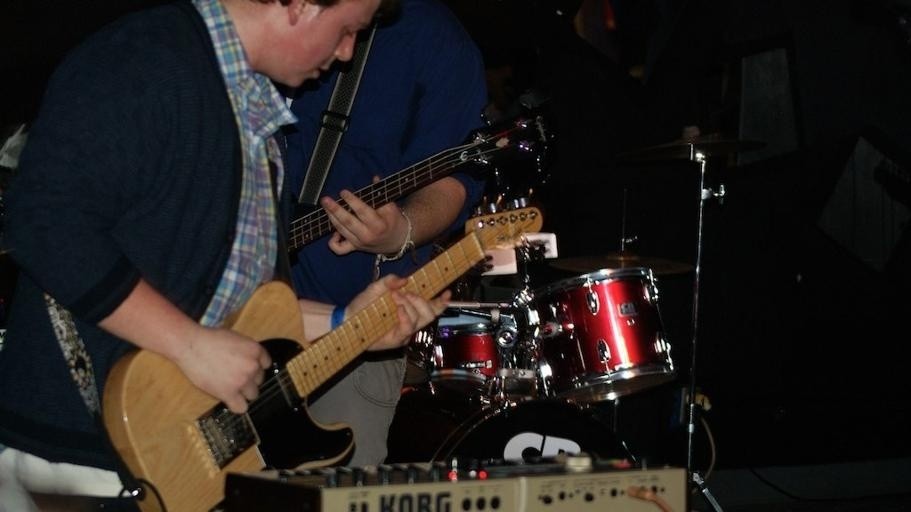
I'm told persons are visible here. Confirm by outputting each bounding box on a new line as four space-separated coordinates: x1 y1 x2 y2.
274 2 486 468
2 1 450 511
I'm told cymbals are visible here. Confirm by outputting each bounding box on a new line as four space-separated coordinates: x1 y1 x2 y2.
549 253 695 276
445 297 542 391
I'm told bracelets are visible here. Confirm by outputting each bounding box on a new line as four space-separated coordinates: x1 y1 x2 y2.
329 306 346 329
374 208 414 281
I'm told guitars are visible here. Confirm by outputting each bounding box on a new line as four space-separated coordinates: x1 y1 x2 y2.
104 183 543 512
286 106 535 254
422 392 638 463
428 313 495 392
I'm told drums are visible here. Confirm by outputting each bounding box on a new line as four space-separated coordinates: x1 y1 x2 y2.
532 265 678 407
